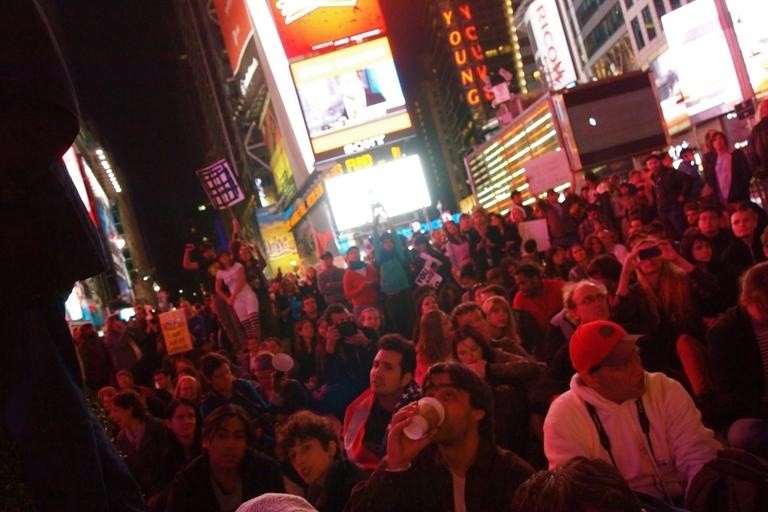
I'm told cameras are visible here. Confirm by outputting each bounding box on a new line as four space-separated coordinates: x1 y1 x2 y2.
638 246 661 260
336 320 358 337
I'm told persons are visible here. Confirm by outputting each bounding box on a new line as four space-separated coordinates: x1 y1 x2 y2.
69 95 768 511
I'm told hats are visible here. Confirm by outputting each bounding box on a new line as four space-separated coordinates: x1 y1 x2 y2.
568 319 646 376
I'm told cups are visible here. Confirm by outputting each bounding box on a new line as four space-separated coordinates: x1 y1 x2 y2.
401 397 444 440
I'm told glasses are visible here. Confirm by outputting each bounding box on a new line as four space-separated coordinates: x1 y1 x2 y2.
574 293 606 306
